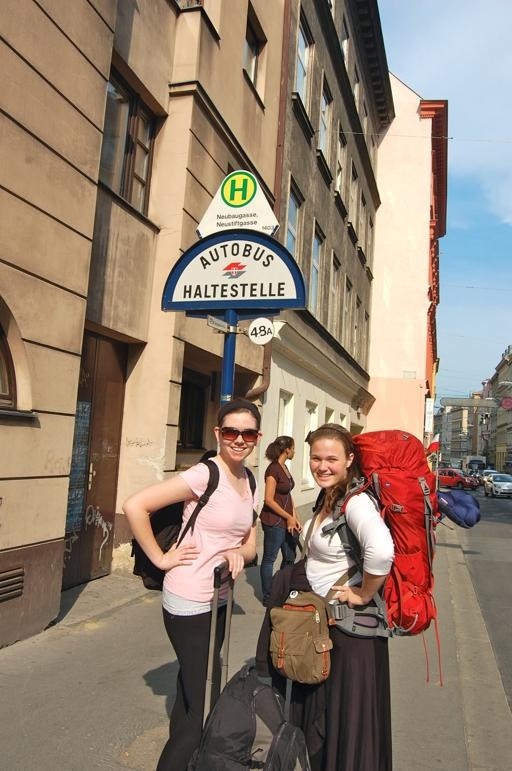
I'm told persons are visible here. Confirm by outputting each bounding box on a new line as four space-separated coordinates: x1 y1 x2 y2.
121 398 261 769
259 435 303 606
257 425 396 771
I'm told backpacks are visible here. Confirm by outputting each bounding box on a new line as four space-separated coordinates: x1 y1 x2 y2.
313 430 481 687
131 451 256 591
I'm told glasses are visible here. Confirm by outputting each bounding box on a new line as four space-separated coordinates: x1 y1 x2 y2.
218 428 258 442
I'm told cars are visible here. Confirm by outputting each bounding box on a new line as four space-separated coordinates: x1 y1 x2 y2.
472 469 512 498
434 468 479 490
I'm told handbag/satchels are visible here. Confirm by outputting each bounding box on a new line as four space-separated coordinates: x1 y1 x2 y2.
270 591 333 684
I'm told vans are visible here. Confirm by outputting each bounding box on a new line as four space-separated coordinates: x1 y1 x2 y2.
438 460 452 468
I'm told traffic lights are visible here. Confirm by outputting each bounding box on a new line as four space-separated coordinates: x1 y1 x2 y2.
479 413 485 424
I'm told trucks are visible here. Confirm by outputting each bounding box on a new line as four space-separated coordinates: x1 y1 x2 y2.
459 456 487 475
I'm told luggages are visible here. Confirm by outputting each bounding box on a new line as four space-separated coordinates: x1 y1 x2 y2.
189 560 312 771
